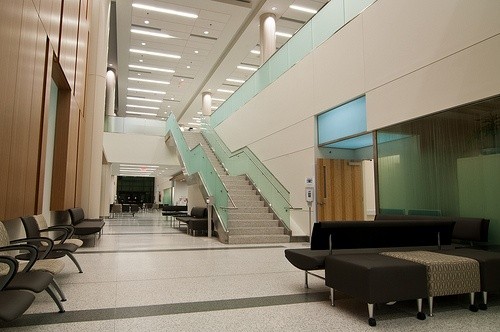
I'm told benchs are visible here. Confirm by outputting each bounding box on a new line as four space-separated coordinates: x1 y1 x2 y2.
110 199 188 221
176 207 207 234
0 221 65 323
188 219 214 236
374 211 492 250
283 218 457 288
67 206 106 240
2 214 83 301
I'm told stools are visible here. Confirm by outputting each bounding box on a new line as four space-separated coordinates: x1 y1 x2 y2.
380 251 481 317
435 247 500 312
324 253 429 325
